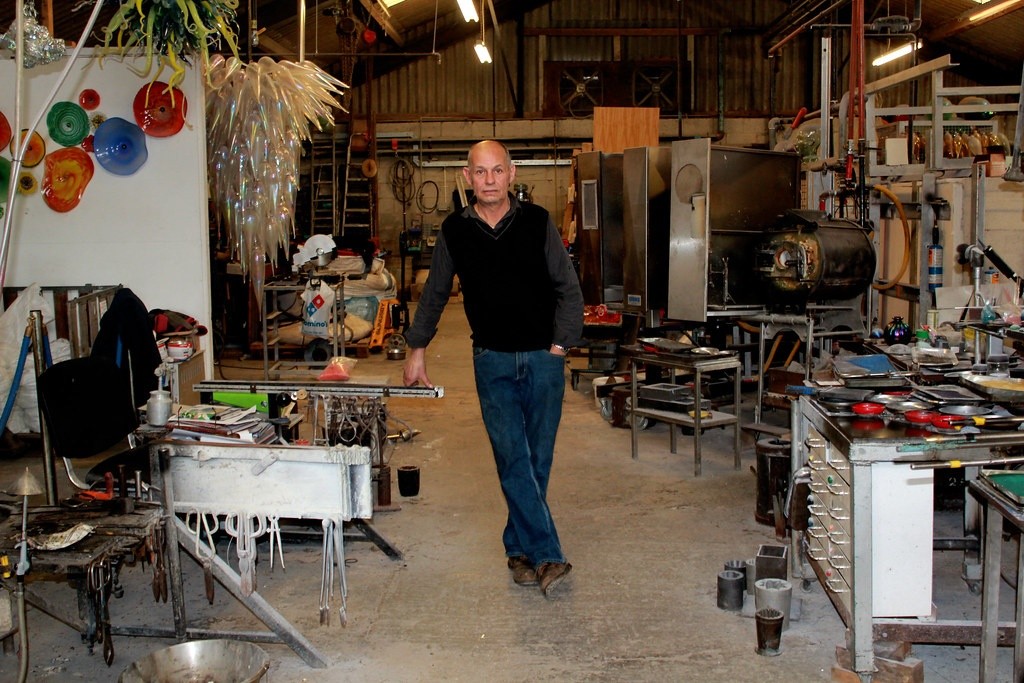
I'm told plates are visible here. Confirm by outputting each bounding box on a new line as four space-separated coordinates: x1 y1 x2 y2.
9 129 45 167
94 117 149 175
46 101 89 147
18 172 38 195
81 135 95 153
79 89 100 109
134 83 188 137
42 146 94 212
0 110 11 151
0 156 11 203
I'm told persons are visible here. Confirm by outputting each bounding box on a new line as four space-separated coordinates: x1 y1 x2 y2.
404 139 584 597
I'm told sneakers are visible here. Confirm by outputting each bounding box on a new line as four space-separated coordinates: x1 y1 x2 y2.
508 557 539 585
535 560 572 600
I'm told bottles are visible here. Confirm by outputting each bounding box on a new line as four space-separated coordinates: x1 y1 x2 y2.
884 316 912 346
981 296 996 324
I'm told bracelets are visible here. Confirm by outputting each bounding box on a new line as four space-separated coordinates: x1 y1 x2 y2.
553 343 569 352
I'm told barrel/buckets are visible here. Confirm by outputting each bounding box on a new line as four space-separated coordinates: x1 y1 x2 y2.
593 377 624 407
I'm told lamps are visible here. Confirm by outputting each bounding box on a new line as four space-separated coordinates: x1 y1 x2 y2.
457 0 480 23
474 0 493 64
871 0 923 67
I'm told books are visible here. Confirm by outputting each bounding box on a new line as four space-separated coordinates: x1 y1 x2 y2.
172 404 278 444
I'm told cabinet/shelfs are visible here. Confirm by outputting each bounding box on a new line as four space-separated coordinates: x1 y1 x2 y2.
166 349 205 406
630 347 742 476
964 476 1024 683
262 280 345 382
789 384 1023 674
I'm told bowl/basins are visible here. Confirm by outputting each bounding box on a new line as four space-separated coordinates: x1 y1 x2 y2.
157 343 167 358
168 342 192 357
120 640 269 683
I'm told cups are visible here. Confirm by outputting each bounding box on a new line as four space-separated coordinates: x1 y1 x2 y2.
987 354 1010 379
